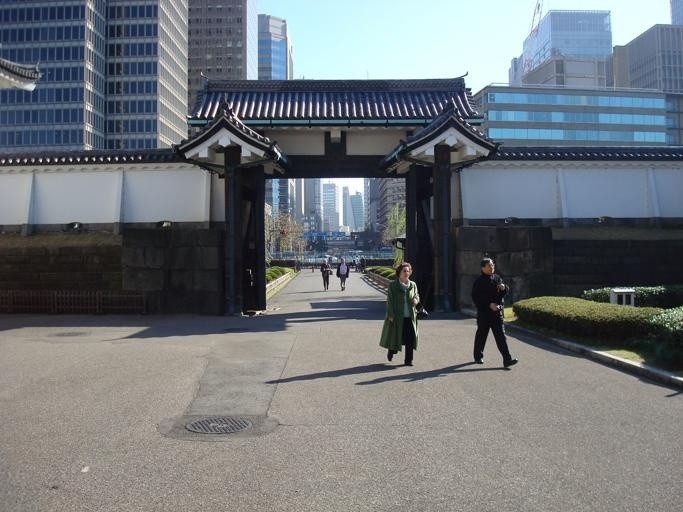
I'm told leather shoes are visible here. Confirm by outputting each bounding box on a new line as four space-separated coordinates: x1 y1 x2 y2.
405 360 412 366
387 350 392 361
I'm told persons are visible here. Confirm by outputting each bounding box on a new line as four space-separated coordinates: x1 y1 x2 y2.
336 257 350 291
355 256 365 272
472 258 518 368
320 259 330 292
379 262 420 366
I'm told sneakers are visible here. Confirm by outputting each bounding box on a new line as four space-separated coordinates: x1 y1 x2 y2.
475 357 483 364
504 358 517 367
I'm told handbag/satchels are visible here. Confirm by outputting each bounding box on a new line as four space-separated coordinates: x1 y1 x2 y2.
416 302 428 320
